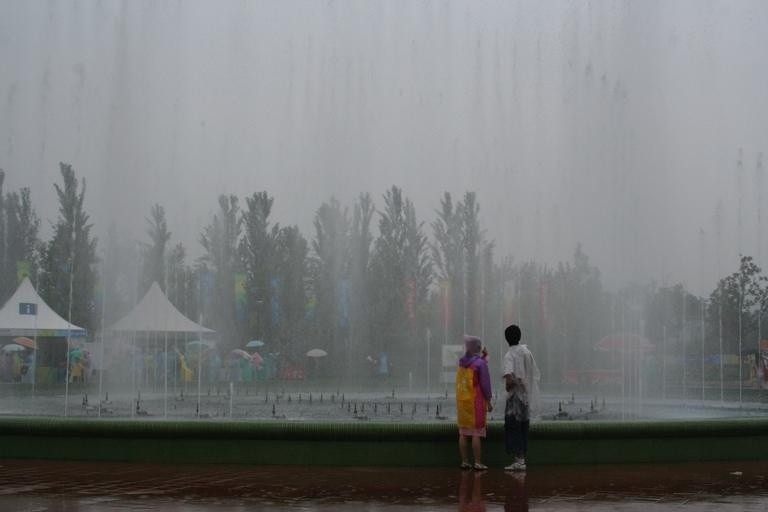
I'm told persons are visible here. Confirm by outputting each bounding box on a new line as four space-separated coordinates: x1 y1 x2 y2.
501 324 541 472
454 335 492 473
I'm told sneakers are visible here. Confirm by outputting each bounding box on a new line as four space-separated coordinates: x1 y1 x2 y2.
460 459 528 471
